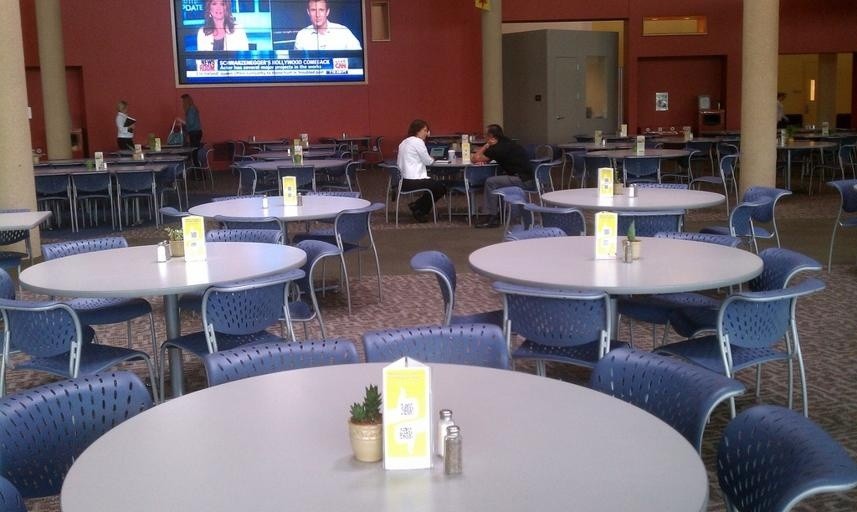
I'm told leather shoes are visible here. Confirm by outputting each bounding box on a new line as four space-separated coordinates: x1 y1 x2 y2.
408 203 428 223
475 215 498 228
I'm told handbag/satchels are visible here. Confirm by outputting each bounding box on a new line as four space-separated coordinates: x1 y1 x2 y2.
167 120 184 145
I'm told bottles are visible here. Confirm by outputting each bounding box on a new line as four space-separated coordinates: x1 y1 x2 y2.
157 239 171 263
159 263 169 279
262 195 269 209
437 407 465 477
628 183 638 197
297 192 303 206
625 241 633 264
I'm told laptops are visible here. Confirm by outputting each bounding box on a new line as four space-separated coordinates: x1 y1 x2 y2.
434 159 452 164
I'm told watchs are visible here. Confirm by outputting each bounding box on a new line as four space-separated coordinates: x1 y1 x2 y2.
485 144 490 149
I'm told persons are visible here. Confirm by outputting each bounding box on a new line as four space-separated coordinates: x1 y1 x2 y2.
199 60 214 70
397 118 447 223
175 94 203 181
116 101 136 150
777 92 788 127
294 0 362 50
471 124 535 227
197 0 249 51
